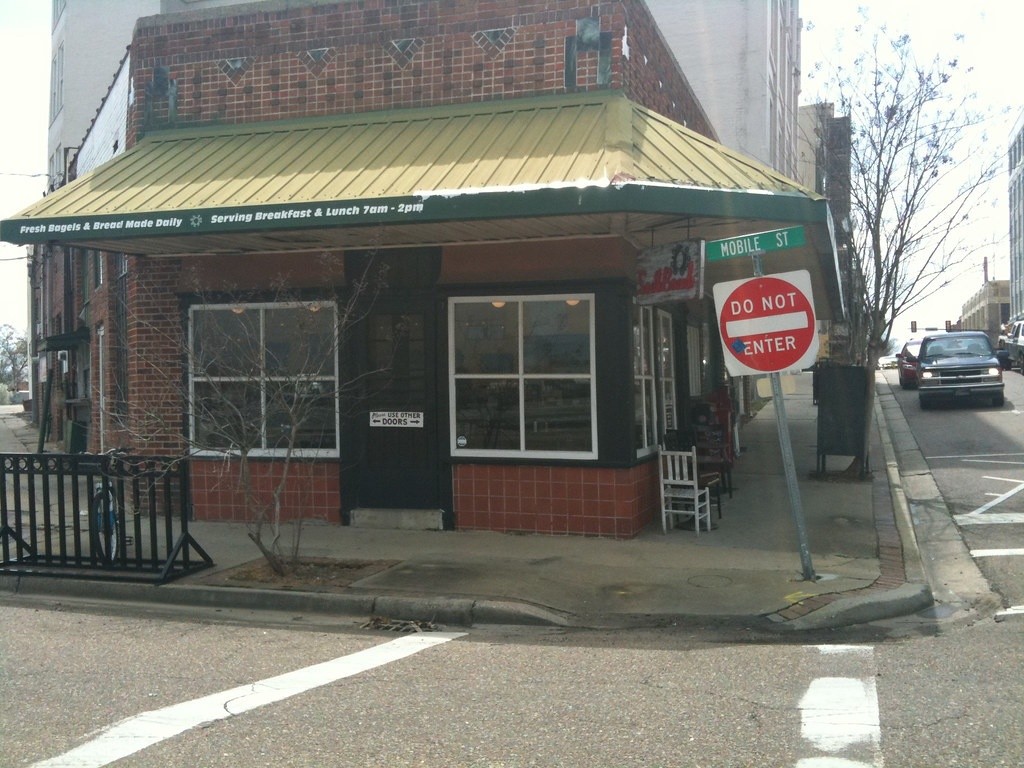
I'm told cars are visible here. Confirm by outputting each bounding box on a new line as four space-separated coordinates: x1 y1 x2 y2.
914 332 1005 408
878 356 898 370
895 340 923 389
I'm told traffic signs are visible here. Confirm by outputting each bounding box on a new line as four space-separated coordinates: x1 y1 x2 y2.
706 225 806 262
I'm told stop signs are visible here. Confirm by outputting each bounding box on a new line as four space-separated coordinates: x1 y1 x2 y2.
713 269 820 377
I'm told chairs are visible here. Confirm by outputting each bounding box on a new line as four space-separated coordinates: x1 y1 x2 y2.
658 444 712 538
690 423 733 499
966 343 983 353
928 346 944 355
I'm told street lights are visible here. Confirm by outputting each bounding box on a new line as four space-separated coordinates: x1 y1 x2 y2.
841 213 856 359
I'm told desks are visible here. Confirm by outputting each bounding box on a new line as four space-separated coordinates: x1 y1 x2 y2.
671 455 725 529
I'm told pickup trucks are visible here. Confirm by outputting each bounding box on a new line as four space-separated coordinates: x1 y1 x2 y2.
997 321 1024 378
